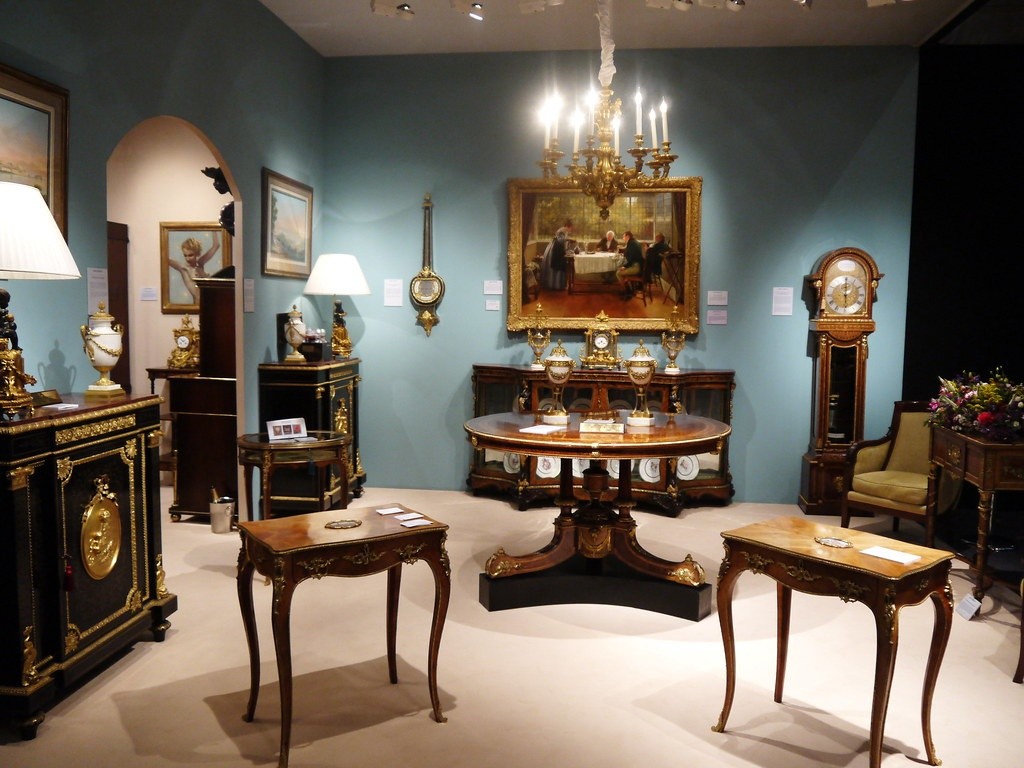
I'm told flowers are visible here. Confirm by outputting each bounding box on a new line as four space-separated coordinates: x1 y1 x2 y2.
932 375 1024 441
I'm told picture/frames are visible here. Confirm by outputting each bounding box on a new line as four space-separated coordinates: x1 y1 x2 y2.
262 167 315 276
0 65 69 243
505 177 703 334
160 221 232 312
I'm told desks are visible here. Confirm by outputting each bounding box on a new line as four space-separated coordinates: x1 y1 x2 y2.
927 422 1024 616
712 516 956 768
238 430 351 518
146 368 198 393
236 502 451 768
464 408 734 621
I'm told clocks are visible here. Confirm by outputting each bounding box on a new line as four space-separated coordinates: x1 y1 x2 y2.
168 314 199 366
581 310 621 370
798 247 884 514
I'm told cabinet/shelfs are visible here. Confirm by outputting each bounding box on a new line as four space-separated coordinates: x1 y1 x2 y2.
258 359 366 513
0 391 177 739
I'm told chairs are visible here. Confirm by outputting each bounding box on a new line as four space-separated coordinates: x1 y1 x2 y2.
841 402 963 546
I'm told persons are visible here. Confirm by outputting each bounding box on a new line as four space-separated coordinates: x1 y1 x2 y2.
333 300 346 327
0 288 23 351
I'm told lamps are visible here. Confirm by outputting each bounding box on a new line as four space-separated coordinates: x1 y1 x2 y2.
302 252 373 359
535 0 680 220
0 182 81 350
